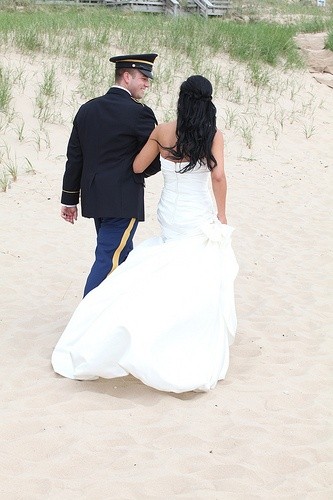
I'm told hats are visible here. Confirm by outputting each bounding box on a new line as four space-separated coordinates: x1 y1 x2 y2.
109 53 158 80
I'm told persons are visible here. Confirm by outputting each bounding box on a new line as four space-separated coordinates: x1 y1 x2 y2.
49 75 236 393
59 53 161 298
